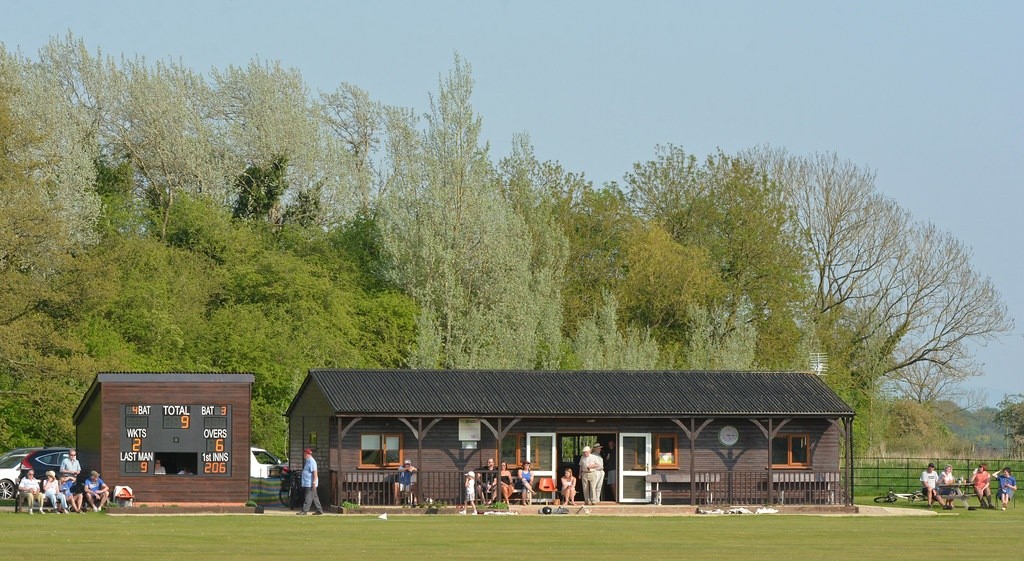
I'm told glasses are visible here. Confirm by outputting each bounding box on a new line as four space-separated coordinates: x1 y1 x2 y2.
71 454 75 456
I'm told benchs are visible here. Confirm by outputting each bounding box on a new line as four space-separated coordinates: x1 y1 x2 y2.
345 475 415 506
770 473 842 504
960 493 979 498
14 478 78 512
644 473 720 508
473 476 533 506
917 494 928 501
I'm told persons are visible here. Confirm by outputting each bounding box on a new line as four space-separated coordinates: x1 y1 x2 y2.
920 463 952 510
392 459 418 506
18 469 109 515
515 460 537 506
155 459 166 474
501 461 514 505
992 467 1017 510
60 450 81 477
459 471 477 515
939 464 954 508
477 459 498 504
970 463 995 509
578 439 616 505
297 448 323 515
561 468 576 505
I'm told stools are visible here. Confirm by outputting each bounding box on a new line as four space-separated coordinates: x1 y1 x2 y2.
561 491 579 505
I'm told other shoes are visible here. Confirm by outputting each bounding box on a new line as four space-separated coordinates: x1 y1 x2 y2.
39 510 46 515
927 505 954 508
471 511 477 515
459 510 467 515
93 507 102 513
296 511 308 516
312 511 323 515
980 504 1007 510
28 511 34 515
585 502 597 505
54 509 85 515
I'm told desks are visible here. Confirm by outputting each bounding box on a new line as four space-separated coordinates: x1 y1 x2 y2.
930 483 975 510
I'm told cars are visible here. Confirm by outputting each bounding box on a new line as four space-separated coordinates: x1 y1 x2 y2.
0 449 69 499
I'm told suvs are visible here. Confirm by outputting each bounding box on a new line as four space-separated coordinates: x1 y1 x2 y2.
19 448 77 492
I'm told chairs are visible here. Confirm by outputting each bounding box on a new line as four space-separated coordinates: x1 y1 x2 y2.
536 478 558 504
81 483 109 512
115 486 136 507
995 493 1015 510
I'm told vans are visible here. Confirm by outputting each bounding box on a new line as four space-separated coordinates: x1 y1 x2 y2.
250 447 282 479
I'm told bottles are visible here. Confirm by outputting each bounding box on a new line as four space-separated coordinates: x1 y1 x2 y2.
958 479 961 483
963 478 966 484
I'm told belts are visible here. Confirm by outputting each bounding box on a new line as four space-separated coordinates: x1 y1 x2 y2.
582 471 593 473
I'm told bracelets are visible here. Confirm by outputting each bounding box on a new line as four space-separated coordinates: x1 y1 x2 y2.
313 482 316 483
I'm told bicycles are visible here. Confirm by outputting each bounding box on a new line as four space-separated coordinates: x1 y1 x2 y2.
279 467 304 510
874 486 928 503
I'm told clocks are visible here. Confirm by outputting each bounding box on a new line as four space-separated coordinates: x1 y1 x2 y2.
718 426 738 446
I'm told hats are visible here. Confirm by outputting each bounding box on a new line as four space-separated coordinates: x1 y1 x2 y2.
403 459 412 465
928 463 935 467
593 442 604 449
464 471 476 478
45 470 57 480
90 471 101 477
583 445 591 453
304 448 313 454
